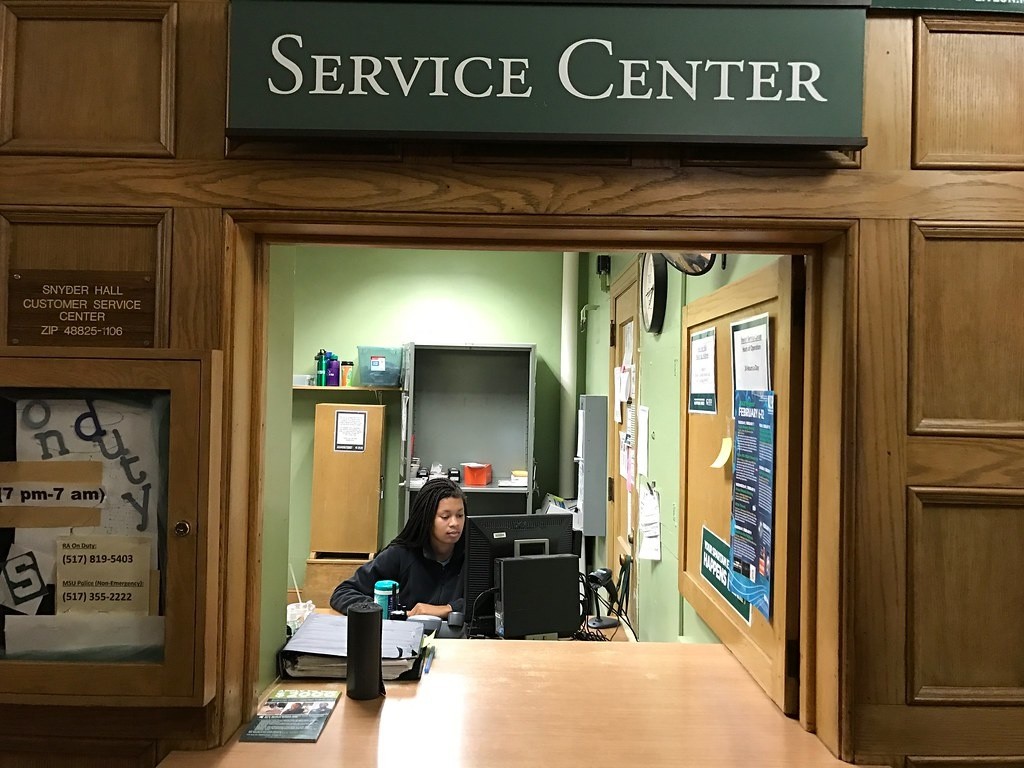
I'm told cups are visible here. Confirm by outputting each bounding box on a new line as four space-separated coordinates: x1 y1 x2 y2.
341 361 354 386
347 603 383 701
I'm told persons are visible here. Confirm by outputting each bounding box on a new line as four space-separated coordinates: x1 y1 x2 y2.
260 702 331 715
330 479 468 619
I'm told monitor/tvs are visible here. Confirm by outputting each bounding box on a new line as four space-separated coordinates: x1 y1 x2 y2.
463 513 573 631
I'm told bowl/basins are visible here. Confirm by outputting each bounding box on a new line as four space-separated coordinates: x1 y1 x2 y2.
293 375 317 387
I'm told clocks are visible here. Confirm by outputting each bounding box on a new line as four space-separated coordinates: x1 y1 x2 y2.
641 252 668 334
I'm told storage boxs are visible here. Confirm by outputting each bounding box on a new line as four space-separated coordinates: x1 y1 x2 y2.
541 493 577 529
463 463 492 486
357 345 402 387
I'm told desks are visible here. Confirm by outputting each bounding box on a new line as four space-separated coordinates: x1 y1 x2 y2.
152 608 856 768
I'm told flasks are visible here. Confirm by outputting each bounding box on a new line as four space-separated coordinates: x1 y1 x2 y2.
374 580 400 619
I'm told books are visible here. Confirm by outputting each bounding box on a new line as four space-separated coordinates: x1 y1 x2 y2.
239 690 342 743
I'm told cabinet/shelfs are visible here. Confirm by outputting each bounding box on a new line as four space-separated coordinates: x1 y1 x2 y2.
398 341 537 529
308 403 386 559
0 346 224 706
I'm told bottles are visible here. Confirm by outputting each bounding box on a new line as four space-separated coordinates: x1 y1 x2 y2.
314 349 340 386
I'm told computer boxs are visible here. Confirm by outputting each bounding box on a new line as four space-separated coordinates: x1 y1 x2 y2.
494 554 581 641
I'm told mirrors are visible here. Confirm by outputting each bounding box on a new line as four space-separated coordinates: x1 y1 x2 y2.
662 254 726 277
